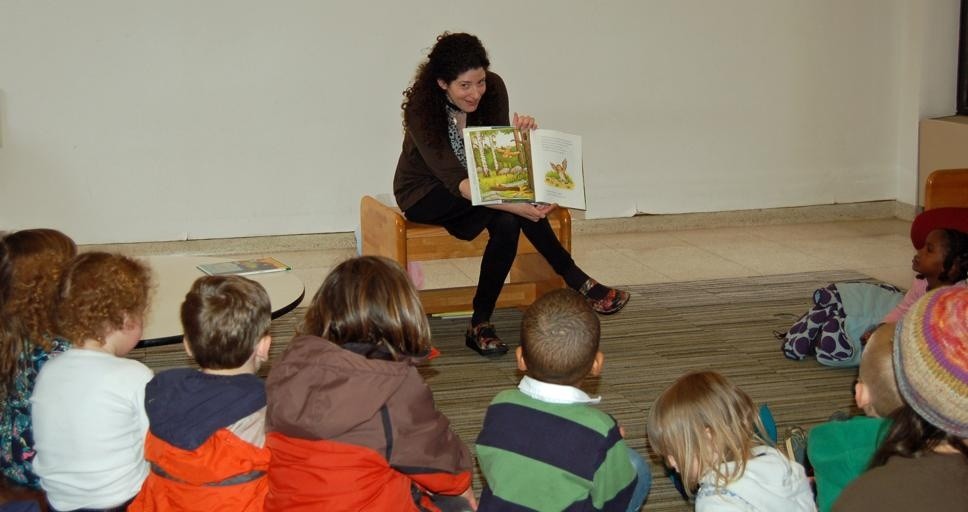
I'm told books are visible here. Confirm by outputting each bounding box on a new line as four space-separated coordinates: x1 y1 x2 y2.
432 311 474 320
197 258 291 274
463 126 587 210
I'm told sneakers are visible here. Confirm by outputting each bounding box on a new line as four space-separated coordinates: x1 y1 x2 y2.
578 279 631 315
466 320 508 356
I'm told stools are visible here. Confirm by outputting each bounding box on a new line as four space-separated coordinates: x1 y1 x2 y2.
360 195 571 318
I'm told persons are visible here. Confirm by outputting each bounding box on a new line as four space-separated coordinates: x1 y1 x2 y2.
475 288 652 512
831 280 968 512
861 207 968 343
648 371 817 512
264 257 478 512
0 229 76 493
807 324 907 512
126 275 273 512
393 30 630 356
29 252 159 512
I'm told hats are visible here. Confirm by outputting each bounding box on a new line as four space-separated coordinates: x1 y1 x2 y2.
893 277 968 437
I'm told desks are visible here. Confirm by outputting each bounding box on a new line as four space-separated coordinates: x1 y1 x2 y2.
132 253 306 348
